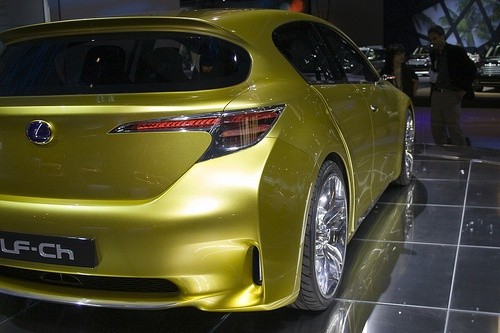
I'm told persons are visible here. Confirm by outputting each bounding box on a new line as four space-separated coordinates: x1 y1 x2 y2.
427 25 479 148
379 43 420 105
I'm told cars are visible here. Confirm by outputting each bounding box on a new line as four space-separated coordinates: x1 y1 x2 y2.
340 41 500 93
0 8 414 311
281 173 428 333
